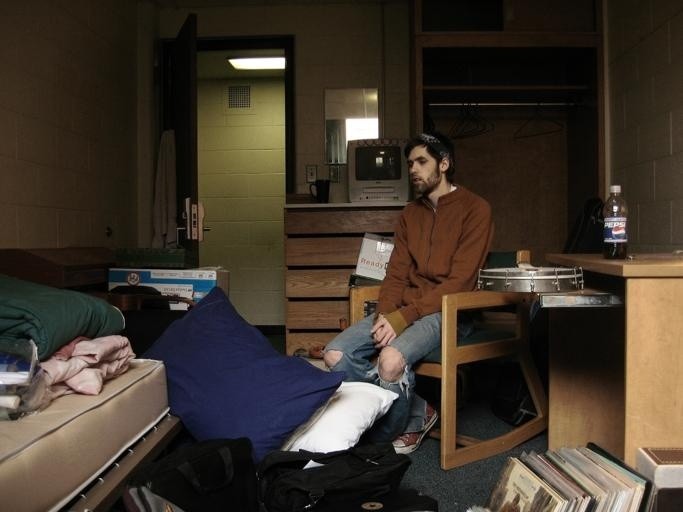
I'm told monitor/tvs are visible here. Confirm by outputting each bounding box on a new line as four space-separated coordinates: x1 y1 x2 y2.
348 140 414 201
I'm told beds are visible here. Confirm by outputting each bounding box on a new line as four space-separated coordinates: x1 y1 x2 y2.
0 273 182 512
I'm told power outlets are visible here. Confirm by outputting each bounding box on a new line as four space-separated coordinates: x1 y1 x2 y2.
305 164 318 183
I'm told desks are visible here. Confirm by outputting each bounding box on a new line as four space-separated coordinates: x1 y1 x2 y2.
546 253 683 473
283 200 410 360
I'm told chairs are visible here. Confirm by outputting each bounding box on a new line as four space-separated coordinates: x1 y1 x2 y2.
349 248 549 472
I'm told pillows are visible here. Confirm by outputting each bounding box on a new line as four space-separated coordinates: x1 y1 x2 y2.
265 381 400 470
136 287 346 472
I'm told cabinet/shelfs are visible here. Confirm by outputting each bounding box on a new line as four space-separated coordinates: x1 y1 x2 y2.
409 0 609 267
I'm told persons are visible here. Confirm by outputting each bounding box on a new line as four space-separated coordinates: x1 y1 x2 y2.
322 131 494 454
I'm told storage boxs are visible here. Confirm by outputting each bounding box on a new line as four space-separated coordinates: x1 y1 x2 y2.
108 268 230 302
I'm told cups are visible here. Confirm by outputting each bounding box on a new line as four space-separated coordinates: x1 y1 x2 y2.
309 179 330 204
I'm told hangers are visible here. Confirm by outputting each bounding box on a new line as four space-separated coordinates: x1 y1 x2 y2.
448 102 563 140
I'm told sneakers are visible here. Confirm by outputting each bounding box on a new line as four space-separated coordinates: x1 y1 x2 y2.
392 402 438 454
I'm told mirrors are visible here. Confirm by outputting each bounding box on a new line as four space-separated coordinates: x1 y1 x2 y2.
324 88 379 165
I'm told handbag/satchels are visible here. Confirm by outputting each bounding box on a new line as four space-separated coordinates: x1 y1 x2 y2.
122 437 258 512
255 436 412 511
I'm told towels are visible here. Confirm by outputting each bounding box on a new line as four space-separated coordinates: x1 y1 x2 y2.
151 130 178 249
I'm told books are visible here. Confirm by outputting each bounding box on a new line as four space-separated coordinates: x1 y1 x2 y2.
486 445 647 512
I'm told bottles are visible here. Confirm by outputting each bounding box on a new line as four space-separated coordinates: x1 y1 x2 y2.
602 184 629 259
